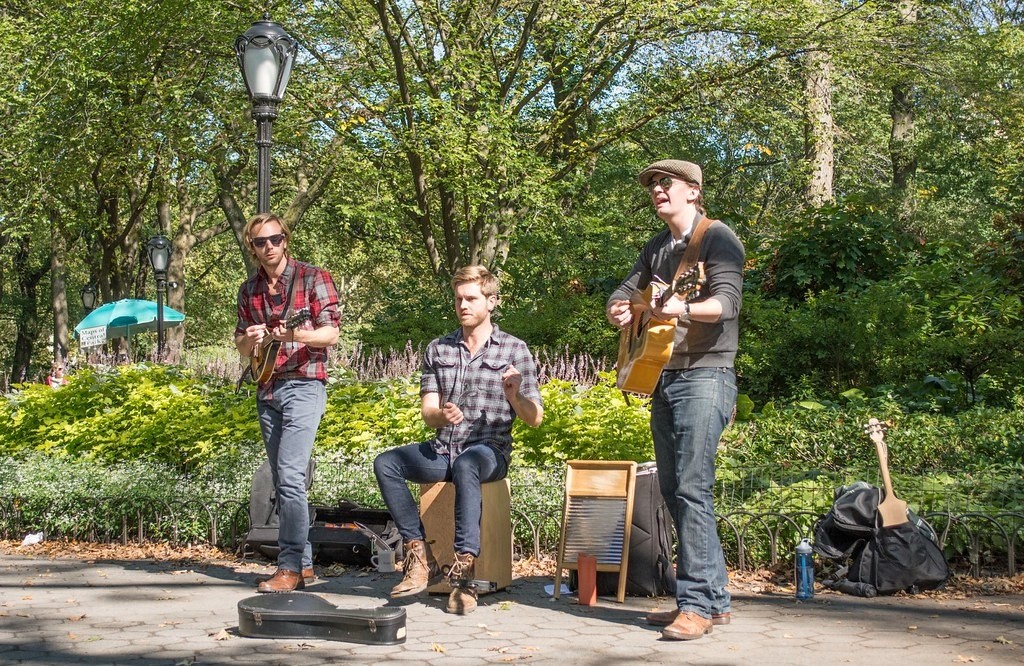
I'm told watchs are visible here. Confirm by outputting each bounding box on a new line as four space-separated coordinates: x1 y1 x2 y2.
677 303 692 320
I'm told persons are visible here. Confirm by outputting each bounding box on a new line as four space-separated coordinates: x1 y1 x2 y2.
375 264 544 614
606 159 747 641
234 213 342 593
18 365 28 383
32 372 39 384
45 362 70 390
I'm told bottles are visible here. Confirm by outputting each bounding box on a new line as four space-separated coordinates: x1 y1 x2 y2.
577 552 597 605
795 539 815 599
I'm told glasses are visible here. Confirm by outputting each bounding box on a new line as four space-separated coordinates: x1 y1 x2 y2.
250 233 285 247
648 177 686 193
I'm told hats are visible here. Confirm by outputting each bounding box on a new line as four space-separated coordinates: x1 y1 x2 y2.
639 159 702 188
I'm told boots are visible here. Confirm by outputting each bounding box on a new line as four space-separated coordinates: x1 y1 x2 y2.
390 539 444 598
447 552 478 614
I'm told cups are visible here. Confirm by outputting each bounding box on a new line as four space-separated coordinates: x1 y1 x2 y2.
371 550 395 573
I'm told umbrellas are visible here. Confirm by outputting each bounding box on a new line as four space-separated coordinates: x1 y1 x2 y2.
72 298 186 366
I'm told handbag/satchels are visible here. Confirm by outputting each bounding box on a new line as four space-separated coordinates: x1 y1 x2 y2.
811 480 950 595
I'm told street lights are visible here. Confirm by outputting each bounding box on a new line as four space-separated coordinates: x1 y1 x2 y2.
233 13 298 213
78 282 98 362
146 232 174 361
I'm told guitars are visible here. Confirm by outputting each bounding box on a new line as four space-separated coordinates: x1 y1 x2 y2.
250 308 312 385
616 261 706 395
863 417 910 526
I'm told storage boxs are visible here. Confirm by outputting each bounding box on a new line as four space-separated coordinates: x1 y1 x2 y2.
420 479 510 595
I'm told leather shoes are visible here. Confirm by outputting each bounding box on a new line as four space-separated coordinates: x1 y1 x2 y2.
662 610 714 640
646 609 731 626
257 568 305 592
300 569 315 584
